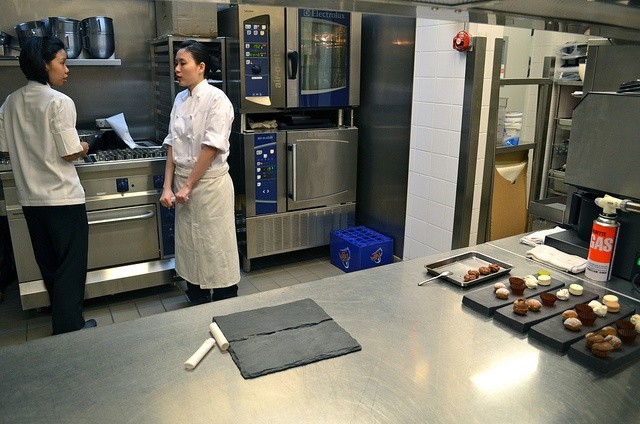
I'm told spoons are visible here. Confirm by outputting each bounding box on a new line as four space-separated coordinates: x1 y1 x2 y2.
417 270 454 286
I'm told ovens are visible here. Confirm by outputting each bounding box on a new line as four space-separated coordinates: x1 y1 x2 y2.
216 3 363 273
0 161 187 314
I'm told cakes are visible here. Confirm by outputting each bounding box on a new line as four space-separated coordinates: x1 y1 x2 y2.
540 292 557 305
515 303 528 314
601 326 617 336
496 287 510 300
525 277 537 289
514 299 527 308
528 298 541 312
523 275 533 284
588 299 602 309
592 304 607 316
569 283 584 296
634 319 640 334
630 313 640 325
509 277 524 289
536 269 550 277
494 282 506 290
604 301 620 312
563 317 582 331
602 294 619 304
615 319 635 329
586 332 603 348
510 283 525 295
538 274 551 285
604 334 622 349
617 327 636 342
575 303 593 315
579 311 598 325
556 288 570 301
479 266 491 275
561 310 578 321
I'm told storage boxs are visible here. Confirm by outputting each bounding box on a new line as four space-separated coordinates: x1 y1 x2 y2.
329 226 394 274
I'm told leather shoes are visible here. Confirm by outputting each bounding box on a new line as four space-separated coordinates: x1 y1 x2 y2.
80 319 97 328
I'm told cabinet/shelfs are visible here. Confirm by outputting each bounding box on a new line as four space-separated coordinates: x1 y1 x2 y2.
542 76 584 199
486 78 553 243
153 37 228 149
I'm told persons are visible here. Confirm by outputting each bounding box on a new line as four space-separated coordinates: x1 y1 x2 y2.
159 39 241 306
0 35 97 335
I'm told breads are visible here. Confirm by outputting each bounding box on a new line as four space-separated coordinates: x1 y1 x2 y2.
467 269 480 279
488 263 500 273
591 342 613 358
463 275 476 282
